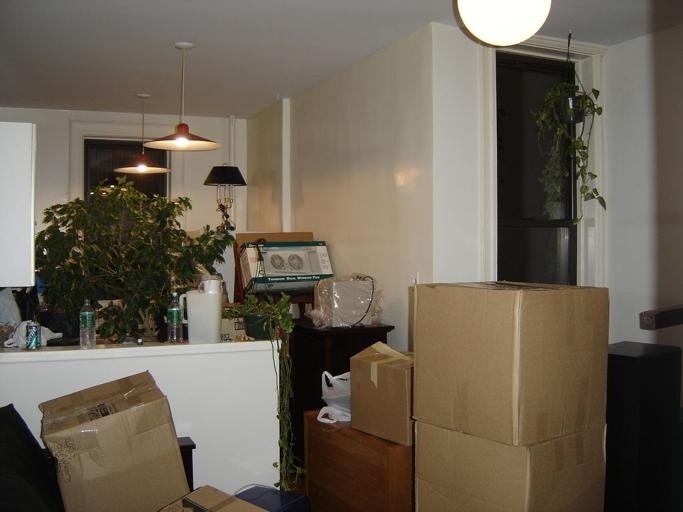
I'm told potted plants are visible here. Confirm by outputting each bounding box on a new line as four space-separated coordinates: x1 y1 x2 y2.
529 83 606 225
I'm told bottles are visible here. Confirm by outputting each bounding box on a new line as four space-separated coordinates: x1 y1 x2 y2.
179 278 222 344
79 298 97 349
167 292 182 341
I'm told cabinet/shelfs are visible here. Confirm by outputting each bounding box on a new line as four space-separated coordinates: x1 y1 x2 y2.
0 340 283 494
291 318 395 469
1 121 36 287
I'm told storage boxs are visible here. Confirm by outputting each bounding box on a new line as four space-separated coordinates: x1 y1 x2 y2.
412 280 609 447
414 421 607 512
349 342 414 448
38 370 192 512
239 240 333 295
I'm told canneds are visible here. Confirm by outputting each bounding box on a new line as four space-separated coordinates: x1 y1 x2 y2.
25 321 42 350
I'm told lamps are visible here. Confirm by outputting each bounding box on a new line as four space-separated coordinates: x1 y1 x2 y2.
143 42 224 151
452 0 552 47
203 165 248 231
112 93 172 174
